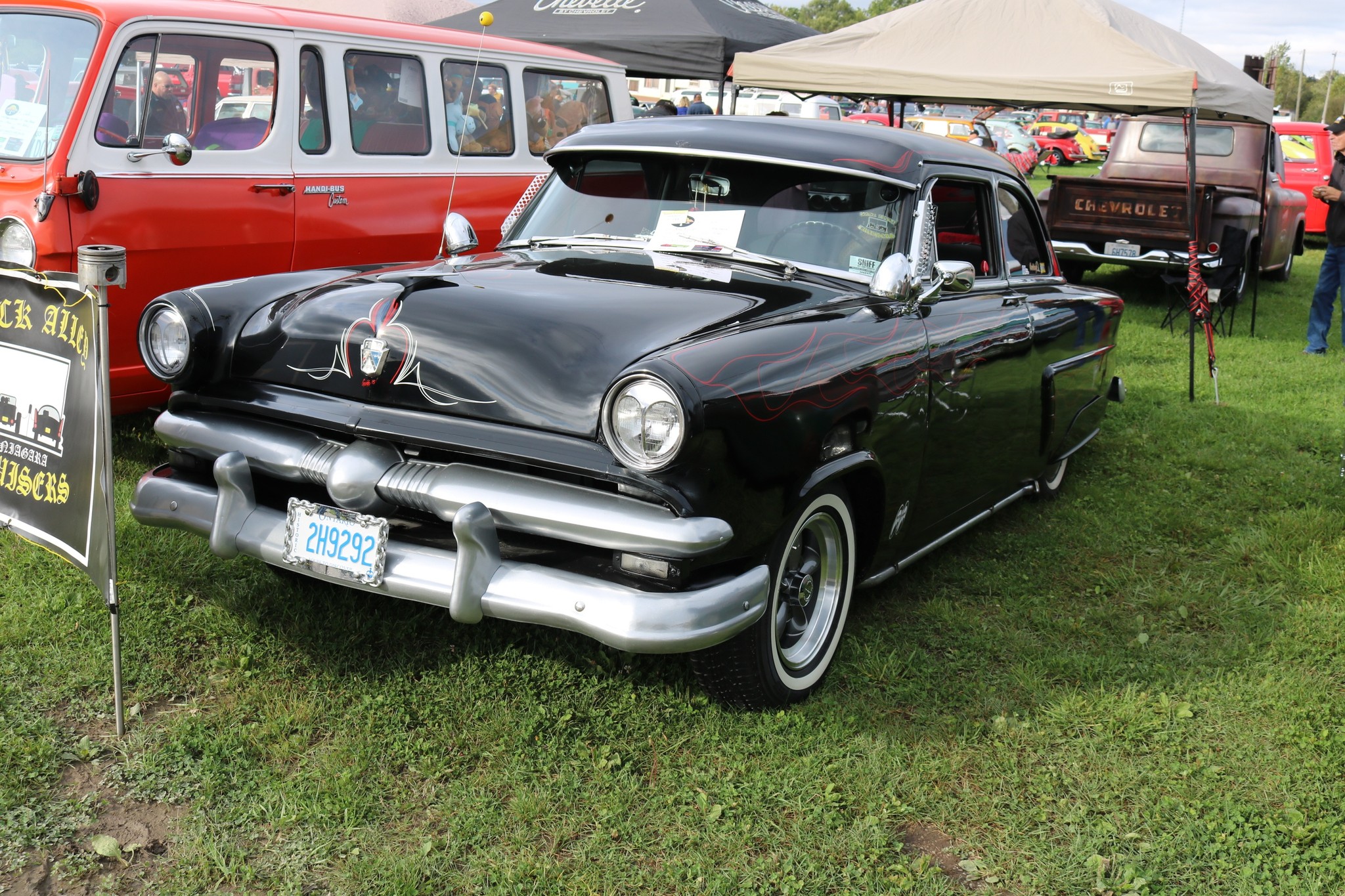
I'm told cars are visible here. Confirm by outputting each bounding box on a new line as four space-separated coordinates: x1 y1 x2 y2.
130 117 1126 714
214 96 311 124
845 102 1125 175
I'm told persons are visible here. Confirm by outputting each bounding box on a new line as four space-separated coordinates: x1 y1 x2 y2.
766 111 789 116
630 93 714 118
863 100 880 113
1300 112 1345 355
488 84 505 109
1000 141 1037 172
934 102 944 113
128 70 188 137
967 130 986 147
914 102 919 111
552 90 567 113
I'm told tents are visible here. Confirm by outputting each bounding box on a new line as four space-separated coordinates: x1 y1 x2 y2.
727 0 1280 409
422 0 825 115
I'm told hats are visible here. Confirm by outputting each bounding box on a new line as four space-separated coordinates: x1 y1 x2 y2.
1323 114 1345 132
655 99 678 115
1026 142 1035 147
970 130 979 134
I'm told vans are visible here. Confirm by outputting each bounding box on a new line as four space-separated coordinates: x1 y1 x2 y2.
670 89 841 123
1 1 635 404
74 66 189 108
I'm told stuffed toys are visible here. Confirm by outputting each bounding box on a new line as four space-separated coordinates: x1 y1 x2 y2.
525 95 589 156
442 61 513 155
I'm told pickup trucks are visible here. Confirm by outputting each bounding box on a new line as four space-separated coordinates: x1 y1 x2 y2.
1036 115 1334 308
144 59 242 98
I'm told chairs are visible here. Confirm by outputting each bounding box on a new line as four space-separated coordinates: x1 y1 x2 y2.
96 112 129 144
1160 225 1248 339
192 117 269 150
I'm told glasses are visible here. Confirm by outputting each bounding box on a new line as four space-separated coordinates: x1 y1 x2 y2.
163 84 174 88
